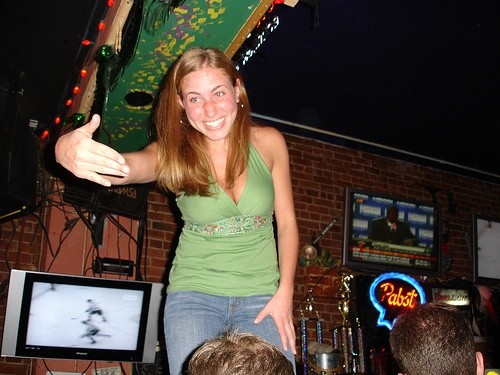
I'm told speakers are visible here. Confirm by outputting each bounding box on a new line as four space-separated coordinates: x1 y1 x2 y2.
0 71 39 221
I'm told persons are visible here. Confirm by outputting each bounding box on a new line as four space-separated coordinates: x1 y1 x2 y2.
184 324 295 375
85 299 107 322
80 320 111 344
55 46 300 375
390 302 485 375
367 204 419 246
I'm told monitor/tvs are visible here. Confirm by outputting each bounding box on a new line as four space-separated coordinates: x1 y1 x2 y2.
1 268 163 363
343 187 442 276
473 214 500 285
